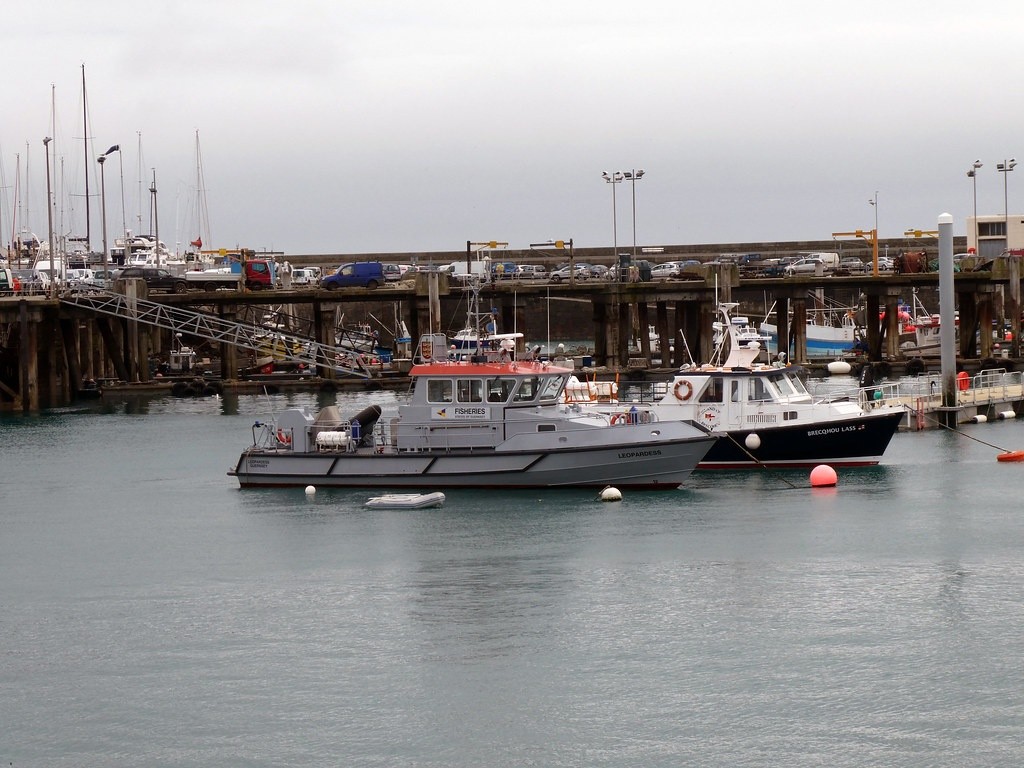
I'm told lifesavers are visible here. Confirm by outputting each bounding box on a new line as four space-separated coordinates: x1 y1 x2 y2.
674 380 693 401
610 413 631 425
278 428 292 442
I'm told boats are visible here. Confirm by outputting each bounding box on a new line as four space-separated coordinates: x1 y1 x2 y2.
227 276 728 489
160 347 220 375
900 287 981 357
449 282 524 349
365 491 445 509
710 303 903 358
557 272 908 467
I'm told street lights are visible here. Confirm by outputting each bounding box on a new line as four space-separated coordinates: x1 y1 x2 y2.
43 137 56 297
602 169 645 281
967 159 1017 255
98 157 109 282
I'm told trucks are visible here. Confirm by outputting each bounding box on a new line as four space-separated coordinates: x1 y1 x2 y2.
447 261 486 279
186 260 273 292
320 260 385 290
804 253 839 271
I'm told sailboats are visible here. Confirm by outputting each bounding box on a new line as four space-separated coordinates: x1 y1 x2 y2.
111 131 169 265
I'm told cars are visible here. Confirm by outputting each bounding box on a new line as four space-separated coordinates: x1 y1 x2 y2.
866 257 898 271
953 254 976 262
589 265 607 278
491 263 546 278
382 264 411 282
650 264 680 278
604 260 650 282
841 257 864 271
783 258 828 275
0 261 189 294
549 266 587 281
